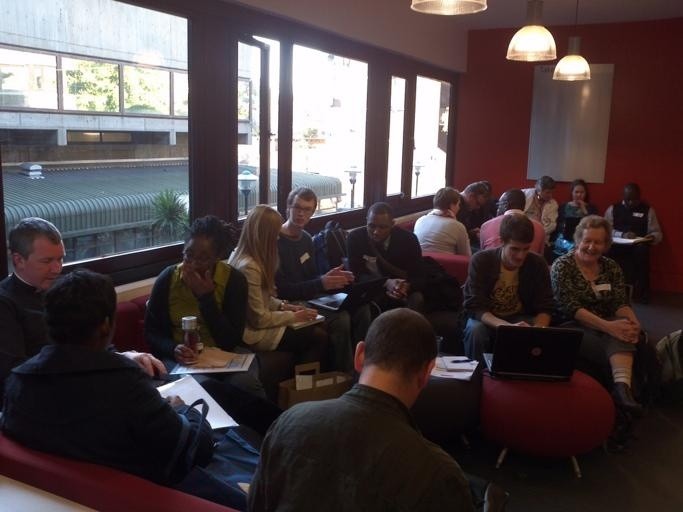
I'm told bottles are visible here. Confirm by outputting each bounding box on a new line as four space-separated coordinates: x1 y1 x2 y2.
555 223 573 253
180 316 198 364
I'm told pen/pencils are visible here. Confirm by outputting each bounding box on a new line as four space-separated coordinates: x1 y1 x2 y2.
451 360 473 363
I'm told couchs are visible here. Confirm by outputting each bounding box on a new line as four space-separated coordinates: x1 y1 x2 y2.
1 217 660 512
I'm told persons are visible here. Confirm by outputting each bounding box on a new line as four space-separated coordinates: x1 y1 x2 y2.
3 270 214 495
241 306 483 511
229 204 329 370
0 216 168 388
140 214 284 432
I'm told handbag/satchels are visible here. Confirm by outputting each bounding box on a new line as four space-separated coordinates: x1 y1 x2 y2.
277 362 355 411
164 399 262 512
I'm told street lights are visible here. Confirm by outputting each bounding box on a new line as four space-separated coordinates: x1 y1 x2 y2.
414 161 424 197
238 166 258 215
342 163 363 210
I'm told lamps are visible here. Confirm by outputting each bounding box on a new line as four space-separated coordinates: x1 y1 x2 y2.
550 1 594 82
504 0 558 63
408 0 489 17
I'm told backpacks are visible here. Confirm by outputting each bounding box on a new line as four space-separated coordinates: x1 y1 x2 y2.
310 219 350 275
650 328 683 409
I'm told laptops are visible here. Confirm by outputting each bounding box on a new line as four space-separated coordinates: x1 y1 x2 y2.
483 324 585 382
307 277 388 314
